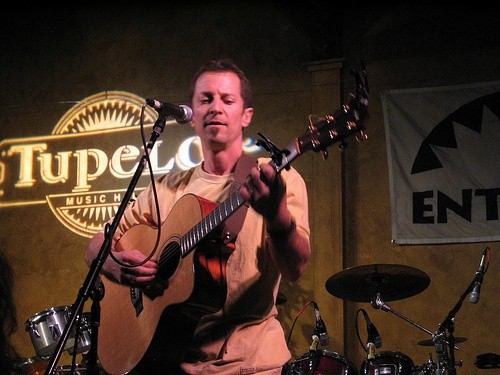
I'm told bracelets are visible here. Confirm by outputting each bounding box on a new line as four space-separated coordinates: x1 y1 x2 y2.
265 216 297 243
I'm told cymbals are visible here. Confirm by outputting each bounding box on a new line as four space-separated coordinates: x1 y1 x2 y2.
324 263 431 304
416 336 470 347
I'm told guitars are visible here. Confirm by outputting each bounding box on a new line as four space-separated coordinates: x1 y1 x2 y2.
93 90 375 375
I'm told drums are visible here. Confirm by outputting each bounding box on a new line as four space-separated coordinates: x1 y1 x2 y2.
359 350 417 375
52 363 87 375
287 349 360 375
23 304 82 358
66 311 93 356
19 355 57 375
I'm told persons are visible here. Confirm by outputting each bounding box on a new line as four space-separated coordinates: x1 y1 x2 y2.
81 59 313 375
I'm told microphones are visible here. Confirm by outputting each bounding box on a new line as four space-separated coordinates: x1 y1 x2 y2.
146 99 193 125
362 309 382 349
470 249 487 304
312 302 329 346
